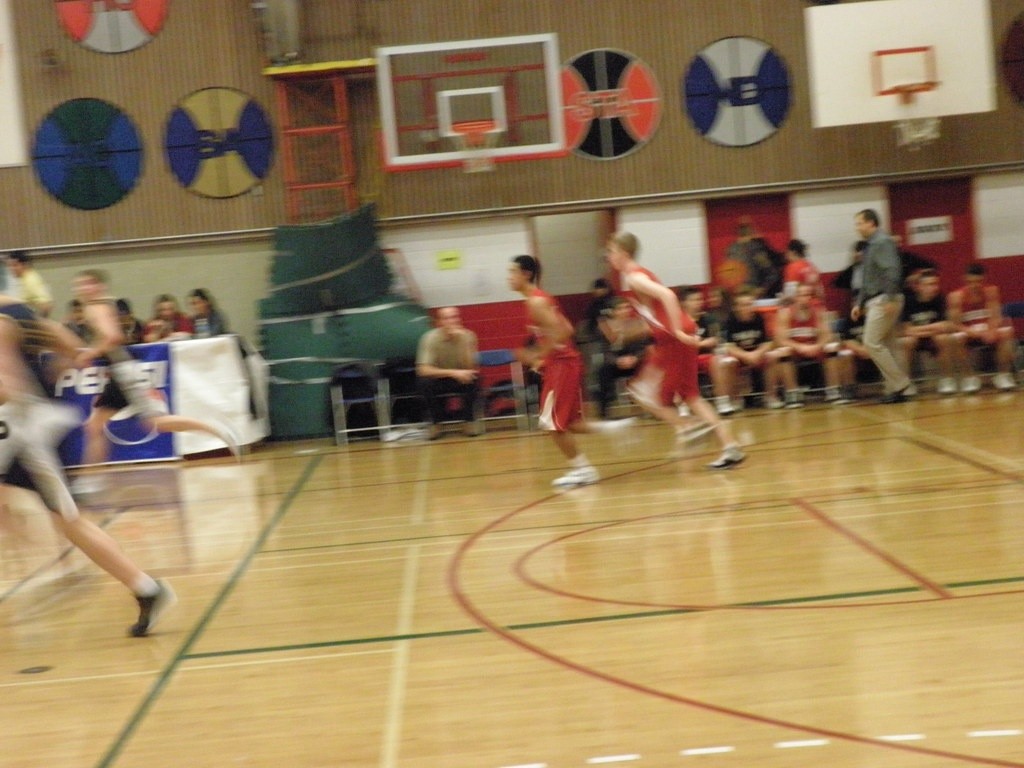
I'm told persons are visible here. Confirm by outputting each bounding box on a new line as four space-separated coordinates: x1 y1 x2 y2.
0 298 180 637
509 254 637 486
851 209 917 403
607 230 747 471
416 307 481 440
680 224 1015 416
517 278 656 421
0 253 242 493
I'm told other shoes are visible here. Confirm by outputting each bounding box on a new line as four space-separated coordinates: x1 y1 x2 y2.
552 466 598 486
938 377 957 394
765 400 785 409
961 376 981 392
705 449 746 470
428 424 443 439
131 579 176 637
825 385 851 405
678 405 690 416
992 373 1017 389
464 420 482 435
732 400 745 413
785 389 804 408
713 395 735 413
876 381 916 403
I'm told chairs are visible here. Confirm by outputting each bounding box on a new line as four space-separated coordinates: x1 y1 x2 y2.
327 347 529 447
575 300 1023 420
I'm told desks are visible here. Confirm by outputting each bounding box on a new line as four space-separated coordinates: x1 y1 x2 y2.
28 332 272 469
